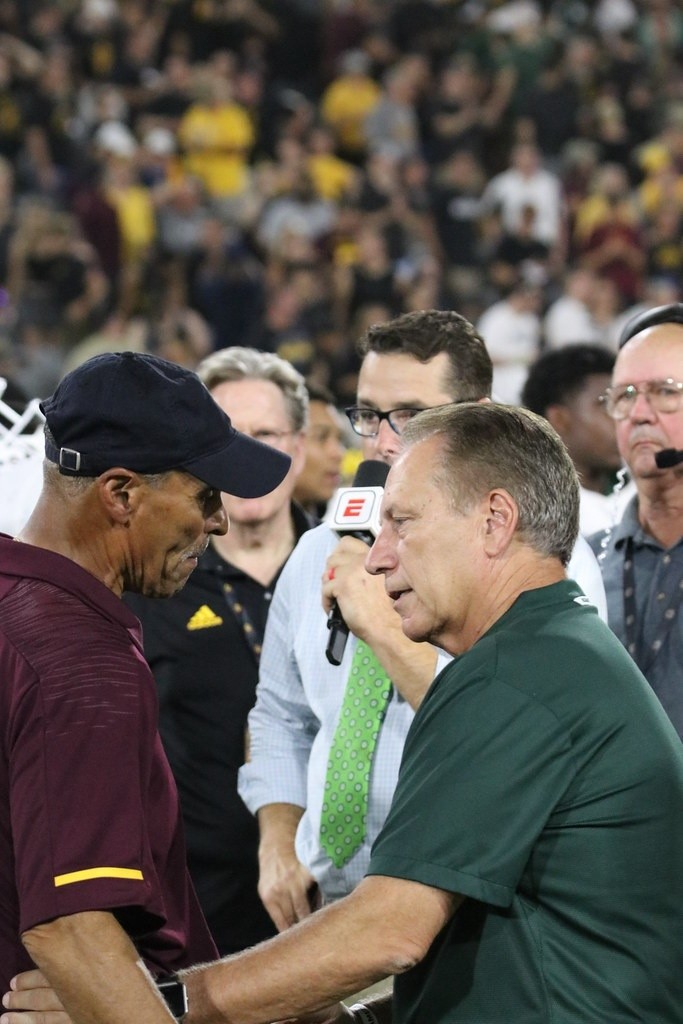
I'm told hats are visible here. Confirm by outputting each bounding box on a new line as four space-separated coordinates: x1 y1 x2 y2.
39 351 291 498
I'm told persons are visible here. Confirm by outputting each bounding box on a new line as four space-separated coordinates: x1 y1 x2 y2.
583 305 683 737
0 350 291 1024
0 402 683 1024
236 306 604 1010
120 344 322 955
0 0 683 561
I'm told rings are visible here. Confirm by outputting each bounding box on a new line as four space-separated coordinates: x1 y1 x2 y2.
328 565 340 580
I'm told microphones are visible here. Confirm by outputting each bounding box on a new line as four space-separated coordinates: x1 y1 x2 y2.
326 460 391 665
654 448 683 467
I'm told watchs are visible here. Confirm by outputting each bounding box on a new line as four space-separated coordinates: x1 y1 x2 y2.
154 968 190 1024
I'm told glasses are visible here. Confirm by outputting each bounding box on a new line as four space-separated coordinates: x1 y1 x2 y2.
247 419 296 446
345 397 479 438
599 378 683 419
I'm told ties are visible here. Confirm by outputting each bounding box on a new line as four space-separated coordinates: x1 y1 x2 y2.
319 633 391 871
